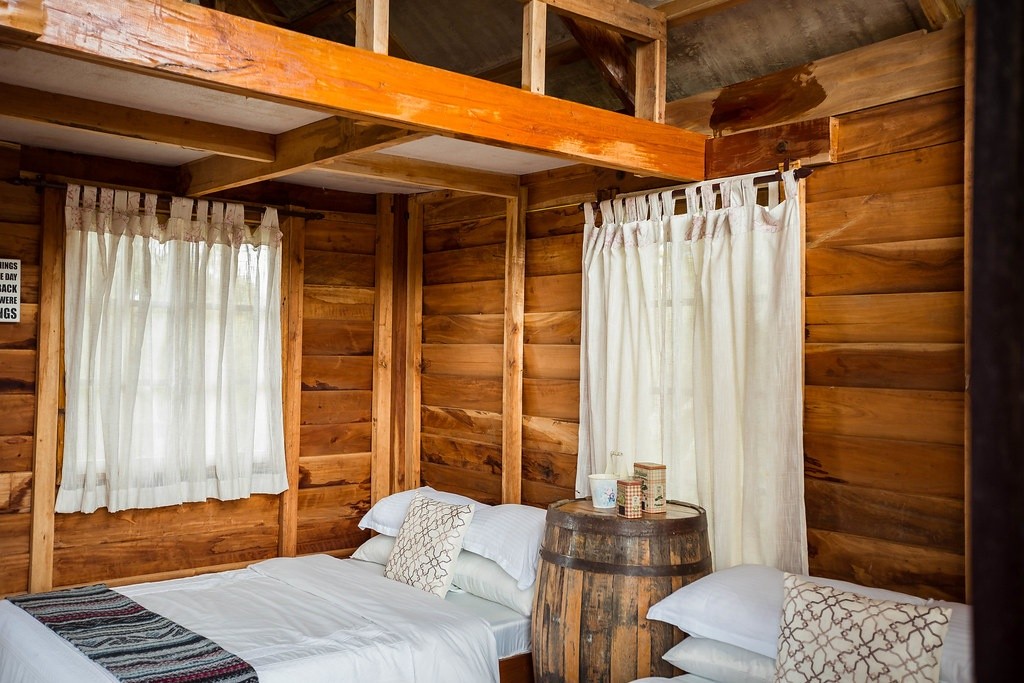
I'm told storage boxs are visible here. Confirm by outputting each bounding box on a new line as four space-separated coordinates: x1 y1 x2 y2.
616 480 642 518
633 462 668 514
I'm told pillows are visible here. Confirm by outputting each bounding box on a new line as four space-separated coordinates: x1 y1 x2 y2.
357 485 492 549
381 490 475 599
772 571 953 683
461 503 548 590
451 548 536 618
645 563 929 662
348 534 468 594
923 597 975 683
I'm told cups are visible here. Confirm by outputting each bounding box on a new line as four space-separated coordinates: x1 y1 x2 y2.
588 473 620 509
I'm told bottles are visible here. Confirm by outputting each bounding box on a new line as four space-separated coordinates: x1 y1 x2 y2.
607 450 629 479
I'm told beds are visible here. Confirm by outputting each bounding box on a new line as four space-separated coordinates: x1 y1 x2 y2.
1 555 534 683
628 674 719 683
